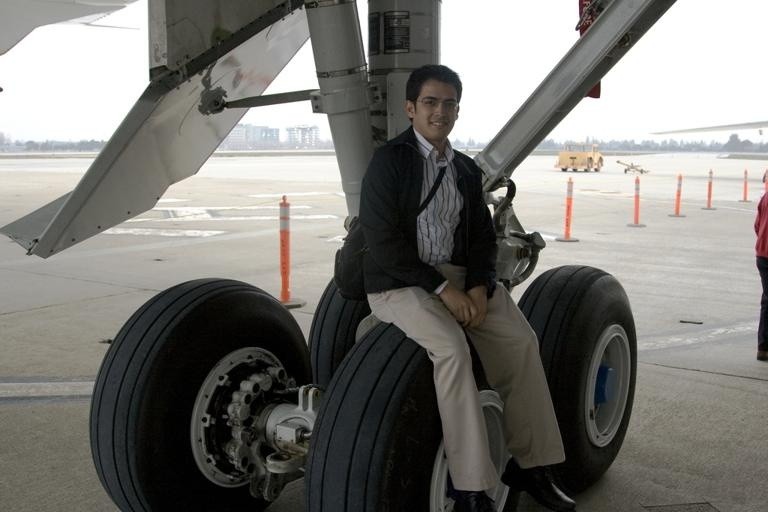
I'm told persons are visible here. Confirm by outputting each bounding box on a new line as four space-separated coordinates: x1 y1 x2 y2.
752 185 768 361
358 63 580 511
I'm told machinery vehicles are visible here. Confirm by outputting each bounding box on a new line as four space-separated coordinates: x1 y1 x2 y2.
553 142 606 174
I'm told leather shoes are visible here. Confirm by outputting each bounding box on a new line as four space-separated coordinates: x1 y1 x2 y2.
500 456 576 511
446 468 496 512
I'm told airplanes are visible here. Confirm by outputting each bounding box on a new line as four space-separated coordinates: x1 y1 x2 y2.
647 117 768 137
0 1 682 512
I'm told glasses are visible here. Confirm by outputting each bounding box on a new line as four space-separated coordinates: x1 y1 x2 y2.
416 98 459 112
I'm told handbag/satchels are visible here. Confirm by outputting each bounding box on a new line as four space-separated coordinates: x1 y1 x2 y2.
334 216 367 301
